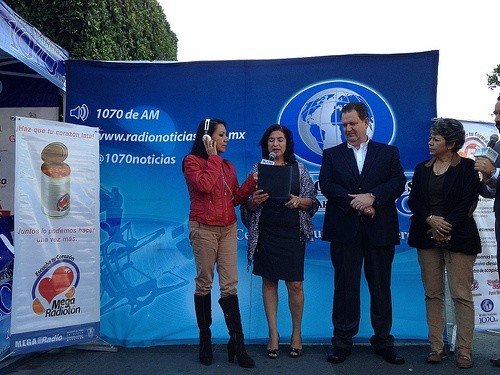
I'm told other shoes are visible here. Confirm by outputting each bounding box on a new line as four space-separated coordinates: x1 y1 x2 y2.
457 356 474 369
427 352 448 362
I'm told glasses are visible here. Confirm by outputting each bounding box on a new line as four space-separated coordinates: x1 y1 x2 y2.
491 113 500 117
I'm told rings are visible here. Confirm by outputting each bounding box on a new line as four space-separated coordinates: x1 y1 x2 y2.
368 209 372 212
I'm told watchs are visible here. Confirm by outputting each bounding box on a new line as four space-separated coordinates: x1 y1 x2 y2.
490 168 496 177
370 192 376 199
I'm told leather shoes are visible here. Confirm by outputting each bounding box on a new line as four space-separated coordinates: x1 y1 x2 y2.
490 357 500 367
373 348 405 365
327 347 352 363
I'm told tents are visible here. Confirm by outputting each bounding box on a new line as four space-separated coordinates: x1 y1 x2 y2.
0 0 69 364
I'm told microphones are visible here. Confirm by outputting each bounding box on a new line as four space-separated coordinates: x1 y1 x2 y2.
474 134 500 181
269 152 276 162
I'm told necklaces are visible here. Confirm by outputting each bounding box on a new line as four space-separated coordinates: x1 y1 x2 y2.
435 158 447 173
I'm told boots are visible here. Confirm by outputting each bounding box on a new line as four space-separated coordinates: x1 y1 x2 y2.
194 292 213 366
217 294 256 369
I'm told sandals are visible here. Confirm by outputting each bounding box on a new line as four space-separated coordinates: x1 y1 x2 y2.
289 334 303 358
266 332 280 359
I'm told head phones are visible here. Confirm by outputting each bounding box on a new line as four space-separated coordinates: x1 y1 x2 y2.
203 119 212 143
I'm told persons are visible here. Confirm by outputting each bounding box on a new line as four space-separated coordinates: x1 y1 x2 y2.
407 118 481 368
319 102 406 364
182 118 259 368
474 96 500 368
241 124 320 358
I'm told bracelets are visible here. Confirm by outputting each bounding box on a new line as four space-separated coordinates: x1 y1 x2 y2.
427 215 433 224
